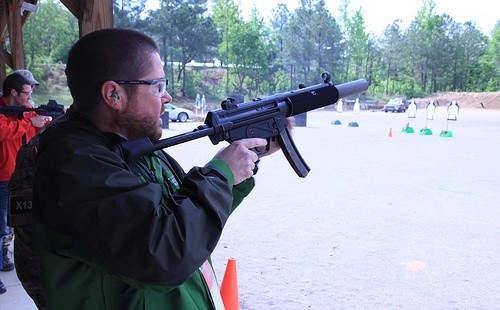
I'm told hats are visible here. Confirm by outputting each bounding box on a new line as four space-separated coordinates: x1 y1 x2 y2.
14 70 40 87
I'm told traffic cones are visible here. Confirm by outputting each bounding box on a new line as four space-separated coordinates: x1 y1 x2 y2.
387 128 393 138
218 255 239 310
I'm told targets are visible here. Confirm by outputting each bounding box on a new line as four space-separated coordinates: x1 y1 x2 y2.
446 99 460 122
337 98 343 114
194 93 201 109
407 98 417 118
352 97 360 116
425 99 436 120
201 95 207 109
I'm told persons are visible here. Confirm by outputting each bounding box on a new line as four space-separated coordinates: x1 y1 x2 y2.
6 28 294 310
0 69 54 295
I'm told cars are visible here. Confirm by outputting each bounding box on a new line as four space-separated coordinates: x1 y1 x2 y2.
161 103 196 123
406 100 417 109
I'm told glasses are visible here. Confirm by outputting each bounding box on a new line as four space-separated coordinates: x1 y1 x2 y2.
115 79 166 96
15 89 31 95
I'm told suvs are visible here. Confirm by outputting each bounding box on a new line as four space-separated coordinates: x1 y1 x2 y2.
383 98 409 113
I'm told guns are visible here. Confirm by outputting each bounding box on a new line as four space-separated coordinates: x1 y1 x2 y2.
112 71 369 178
0 99 69 121
